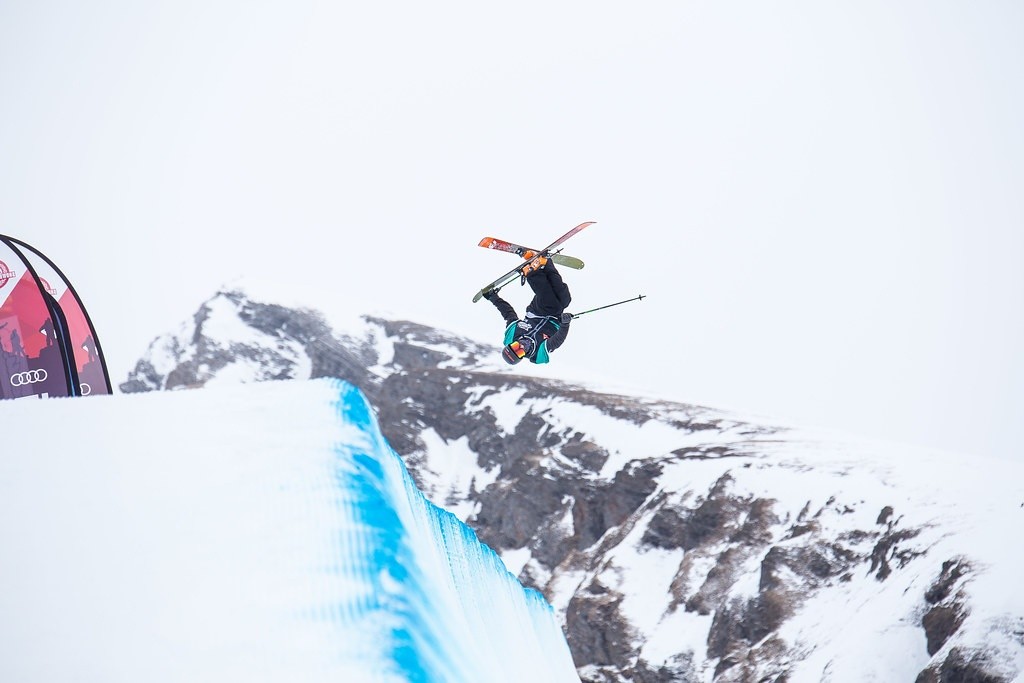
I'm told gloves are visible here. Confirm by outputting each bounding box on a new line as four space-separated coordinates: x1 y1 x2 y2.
558 312 573 326
482 288 500 304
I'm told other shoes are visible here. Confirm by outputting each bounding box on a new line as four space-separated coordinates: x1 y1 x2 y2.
521 256 547 277
523 251 537 260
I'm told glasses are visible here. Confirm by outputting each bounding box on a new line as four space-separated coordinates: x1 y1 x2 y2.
509 341 526 359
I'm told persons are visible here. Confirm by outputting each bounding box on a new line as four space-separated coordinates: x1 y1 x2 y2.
483 248 572 365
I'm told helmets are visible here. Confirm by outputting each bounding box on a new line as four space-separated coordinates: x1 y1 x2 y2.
502 343 524 366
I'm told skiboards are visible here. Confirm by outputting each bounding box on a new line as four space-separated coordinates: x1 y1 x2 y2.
477 237 584 270
472 221 597 302
